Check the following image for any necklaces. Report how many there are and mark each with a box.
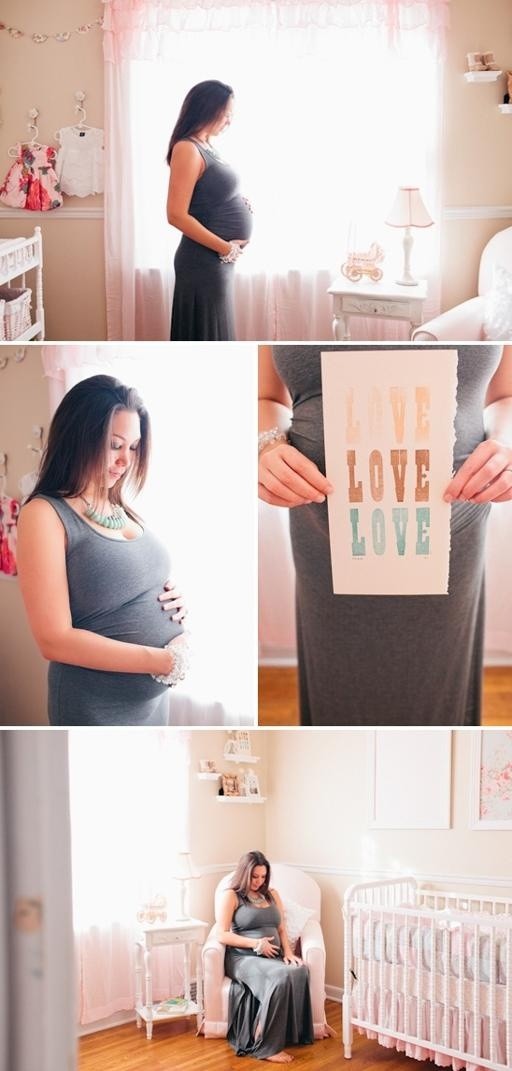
[246,892,267,905]
[77,487,129,530]
[194,134,224,161]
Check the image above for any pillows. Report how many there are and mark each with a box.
[280,892,315,953]
[483,261,512,340]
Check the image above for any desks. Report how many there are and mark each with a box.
[126,917,208,1040]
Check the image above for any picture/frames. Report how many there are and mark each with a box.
[367,731,454,828]
[469,730,512,832]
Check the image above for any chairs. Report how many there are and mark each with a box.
[411,219,512,340]
[201,863,329,1040]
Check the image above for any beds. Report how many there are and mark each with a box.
[339,875,512,1070]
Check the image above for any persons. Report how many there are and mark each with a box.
[257,345,512,726]
[14,372,190,726]
[164,76,254,341]
[215,850,311,1064]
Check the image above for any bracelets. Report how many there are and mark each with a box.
[256,423,283,449]
[149,642,186,686]
[252,939,265,955]
[217,242,244,267]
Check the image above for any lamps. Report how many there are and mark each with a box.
[385,183,437,287]
[170,852,200,920]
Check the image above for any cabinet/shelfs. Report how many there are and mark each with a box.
[463,69,512,115]
[0,225,44,342]
[325,281,430,340]
[197,753,268,804]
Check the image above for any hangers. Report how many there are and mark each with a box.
[53,106,91,141]
[0,472,19,515]
[17,446,43,491]
[6,125,56,159]
